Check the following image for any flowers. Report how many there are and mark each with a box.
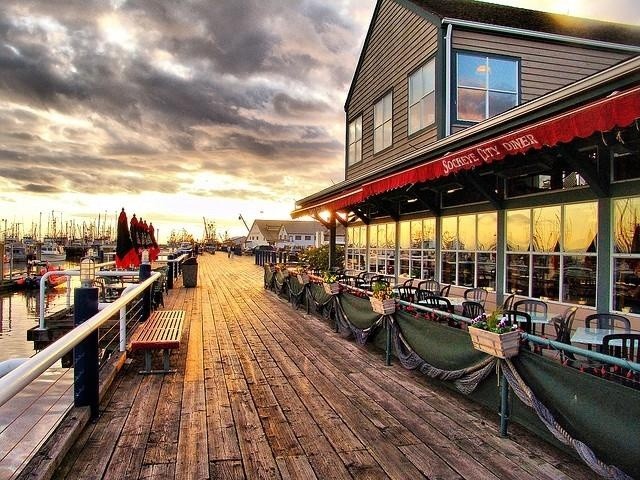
[298,268,304,274]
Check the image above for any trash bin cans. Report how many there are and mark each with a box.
[181,258,198,288]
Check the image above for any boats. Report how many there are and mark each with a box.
[0,210,117,295]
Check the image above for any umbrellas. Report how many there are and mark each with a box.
[116,207,160,287]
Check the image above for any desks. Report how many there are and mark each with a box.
[569,325,639,352]
[418,297,481,306]
[496,310,550,333]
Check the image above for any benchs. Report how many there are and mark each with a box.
[132,309,185,375]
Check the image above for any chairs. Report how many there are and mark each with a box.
[512,298,549,336]
[540,306,591,370]
[301,263,454,315]
[583,312,631,353]
[600,333,640,384]
[501,309,533,341]
[460,300,486,321]
[501,294,514,311]
[464,287,489,308]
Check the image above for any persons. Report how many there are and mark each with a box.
[27,249,36,276]
[271,243,276,251]
[227,244,236,259]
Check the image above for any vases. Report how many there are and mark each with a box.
[296,274,309,285]
